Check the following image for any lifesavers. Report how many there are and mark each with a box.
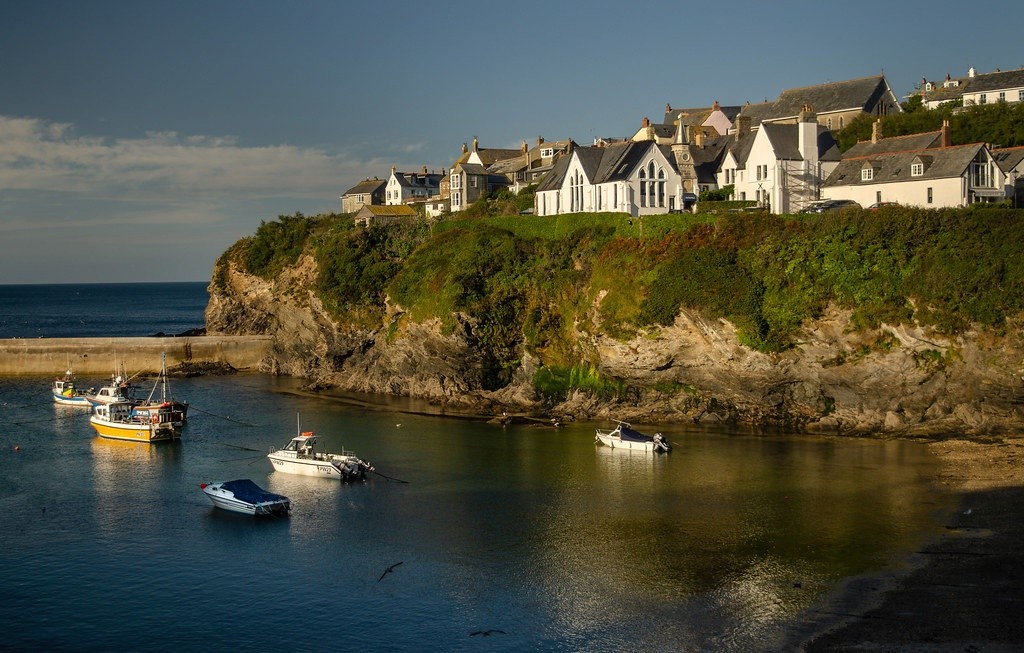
[301,431,314,436]
[151,413,158,424]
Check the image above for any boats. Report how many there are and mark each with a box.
[202,477,292,516]
[90,352,188,442]
[52,351,96,406]
[85,349,131,406]
[105,349,130,396]
[595,418,672,452]
[268,412,375,481]
